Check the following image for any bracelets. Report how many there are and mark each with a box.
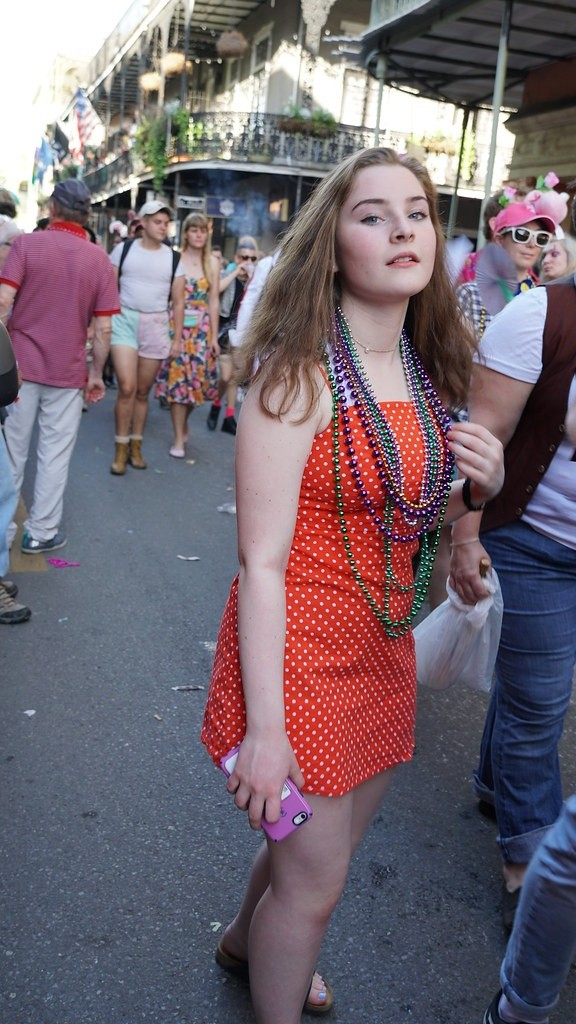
[462,478,482,511]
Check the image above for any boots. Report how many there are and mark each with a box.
[110,435,130,474]
[129,433,147,469]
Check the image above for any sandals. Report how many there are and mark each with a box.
[213,928,334,1013]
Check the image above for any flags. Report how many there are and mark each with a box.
[76,87,105,147]
[32,121,71,184]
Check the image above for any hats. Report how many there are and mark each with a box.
[492,185,556,245]
[53,180,90,211]
[139,201,176,221]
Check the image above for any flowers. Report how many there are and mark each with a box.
[497,172,558,213]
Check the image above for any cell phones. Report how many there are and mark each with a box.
[220,746,312,842]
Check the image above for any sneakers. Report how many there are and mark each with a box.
[21,531,67,553]
[0,578,32,624]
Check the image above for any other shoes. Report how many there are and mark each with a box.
[82,402,89,412]
[169,443,185,458]
[102,375,117,390]
[221,417,237,436]
[159,396,170,410]
[206,404,222,430]
[500,868,521,933]
[482,988,531,1024]
[476,797,498,824]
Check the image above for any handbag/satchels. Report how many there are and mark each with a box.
[411,564,504,693]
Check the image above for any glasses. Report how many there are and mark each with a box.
[497,225,552,249]
[237,254,257,262]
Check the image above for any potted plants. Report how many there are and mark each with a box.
[281,105,338,138]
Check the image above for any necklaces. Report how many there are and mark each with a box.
[314,308,459,639]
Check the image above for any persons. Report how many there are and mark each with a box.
[203,144,507,1017]
[453,177,576,959]
[0,179,121,552]
[0,189,279,475]
[1,316,32,626]
